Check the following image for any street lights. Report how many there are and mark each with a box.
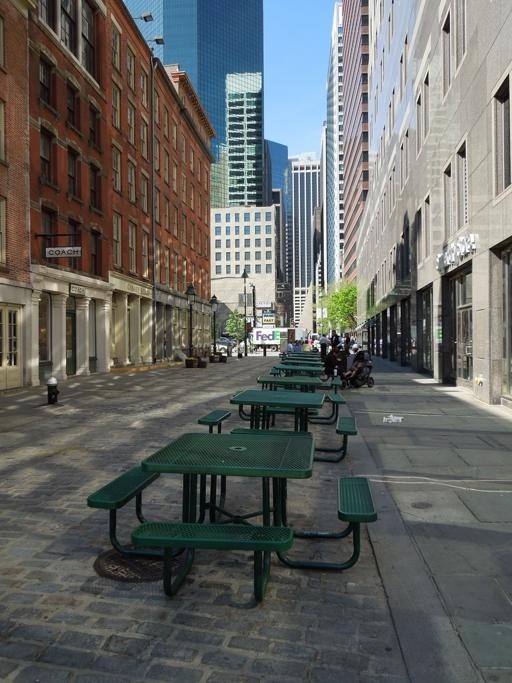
[240,268,251,356]
[184,282,197,358]
[209,294,219,356]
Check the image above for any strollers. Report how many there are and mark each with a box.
[340,350,376,389]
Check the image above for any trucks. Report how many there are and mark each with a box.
[253,328,318,351]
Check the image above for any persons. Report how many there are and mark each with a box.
[287,330,364,380]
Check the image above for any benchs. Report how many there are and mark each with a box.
[198,407,232,434]
[86,431,204,546]
[132,522,291,602]
[283,375,378,569]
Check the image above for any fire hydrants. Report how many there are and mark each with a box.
[47,377,60,405]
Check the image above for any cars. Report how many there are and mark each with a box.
[217,337,234,352]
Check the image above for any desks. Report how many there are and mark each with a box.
[231,388,325,436]
[258,376,323,392]
[274,352,326,372]
[144,432,316,525]
